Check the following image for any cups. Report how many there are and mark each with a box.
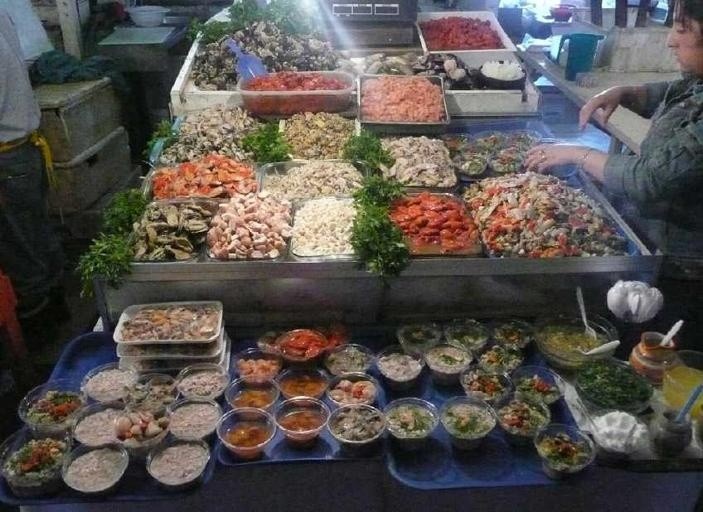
[661,351,703,416]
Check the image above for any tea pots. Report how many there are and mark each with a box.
[626,330,675,386]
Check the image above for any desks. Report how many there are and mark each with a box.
[515,39,682,157]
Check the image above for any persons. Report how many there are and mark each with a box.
[521,0,701,312]
[0,0,76,361]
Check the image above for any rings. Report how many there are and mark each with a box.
[537,150,545,154]
[538,155,550,162]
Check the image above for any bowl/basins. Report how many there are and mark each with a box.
[219,328,383,458]
[573,357,655,415]
[443,319,492,351]
[536,311,619,368]
[382,397,439,440]
[550,5,576,22]
[121,5,171,28]
[510,365,568,405]
[0,359,231,493]
[587,408,647,455]
[427,341,474,377]
[459,362,510,399]
[442,126,579,182]
[493,316,536,351]
[533,425,597,480]
[395,321,443,355]
[498,391,553,442]
[377,345,426,381]
[439,395,498,441]
[474,347,526,374]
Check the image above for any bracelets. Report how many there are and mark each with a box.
[577,144,592,170]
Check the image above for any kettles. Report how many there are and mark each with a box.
[554,34,606,83]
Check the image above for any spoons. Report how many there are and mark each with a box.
[574,286,597,342]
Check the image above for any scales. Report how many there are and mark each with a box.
[327,1,416,48]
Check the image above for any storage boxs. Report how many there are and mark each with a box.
[97,26,176,72]
[32,77,143,240]
